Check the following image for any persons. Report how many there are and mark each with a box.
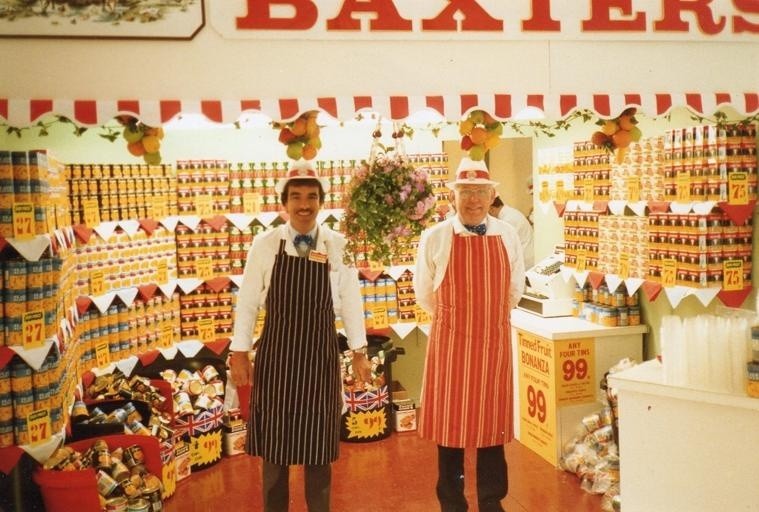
[412,159,526,512]
[487,192,535,273]
[231,162,372,511]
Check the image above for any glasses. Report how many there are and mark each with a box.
[459,188,491,198]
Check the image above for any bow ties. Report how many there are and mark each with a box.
[464,223,486,236]
[294,234,314,248]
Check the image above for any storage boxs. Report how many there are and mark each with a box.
[393,399,416,432]
[392,380,407,399]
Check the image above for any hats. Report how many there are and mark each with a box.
[445,157,500,188]
[275,164,330,195]
[490,192,499,206]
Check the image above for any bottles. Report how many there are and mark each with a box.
[48,157,65,193]
[5,262,27,347]
[15,194,31,203]
[46,341,84,434]
[43,196,71,233]
[681,319,708,388]
[180,160,228,211]
[64,164,178,225]
[727,319,747,392]
[11,363,34,445]
[231,162,368,209]
[564,122,759,302]
[157,365,225,418]
[660,316,683,378]
[175,222,398,340]
[56,230,179,378]
[398,157,454,319]
[557,357,636,494]
[31,193,48,234]
[0,370,14,447]
[572,270,641,327]
[0,193,15,238]
[11,151,31,193]
[43,439,163,512]
[0,150,14,192]
[710,318,727,391]
[72,372,176,441]
[30,152,49,192]
[39,259,55,337]
[340,349,385,392]
[24,262,43,310]
[26,364,51,409]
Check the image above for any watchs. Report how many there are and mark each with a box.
[353,346,369,355]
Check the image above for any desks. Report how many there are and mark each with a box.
[509,308,650,468]
[607,356,759,512]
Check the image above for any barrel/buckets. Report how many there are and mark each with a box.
[72,401,151,434]
[340,335,406,443]
[81,380,175,500]
[33,435,162,512]
[121,358,226,472]
